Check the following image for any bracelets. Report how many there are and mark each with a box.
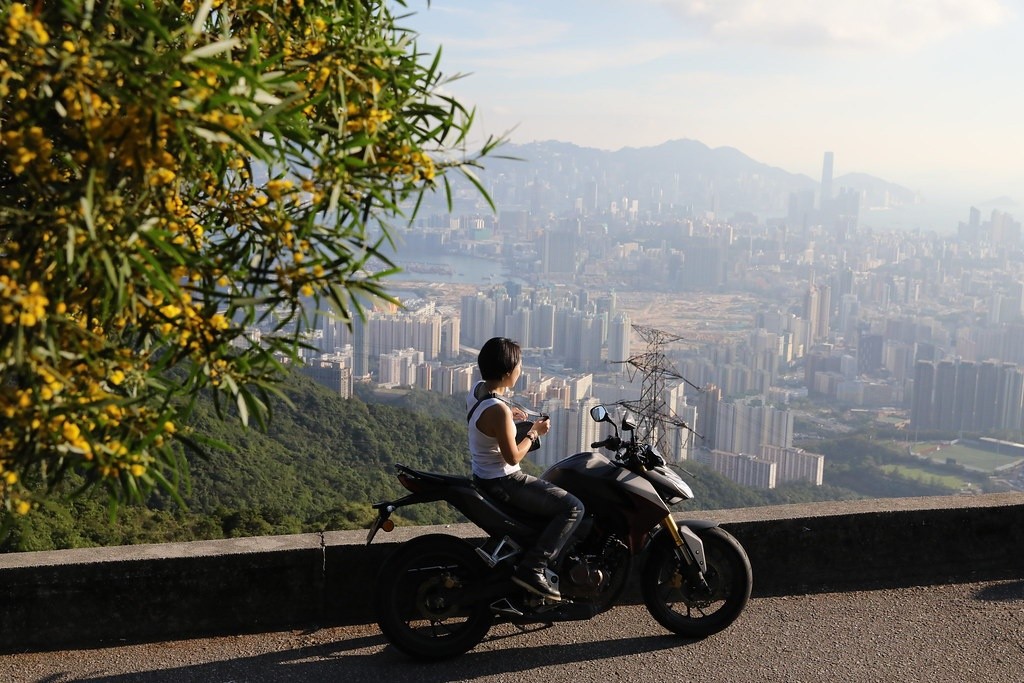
[527,430,538,440]
[526,434,533,440]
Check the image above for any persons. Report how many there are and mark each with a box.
[466,337,585,600]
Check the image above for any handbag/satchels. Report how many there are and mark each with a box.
[515,420,540,453]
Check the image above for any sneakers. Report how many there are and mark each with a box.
[511,564,562,600]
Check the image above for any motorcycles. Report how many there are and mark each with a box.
[366,405,753,663]
